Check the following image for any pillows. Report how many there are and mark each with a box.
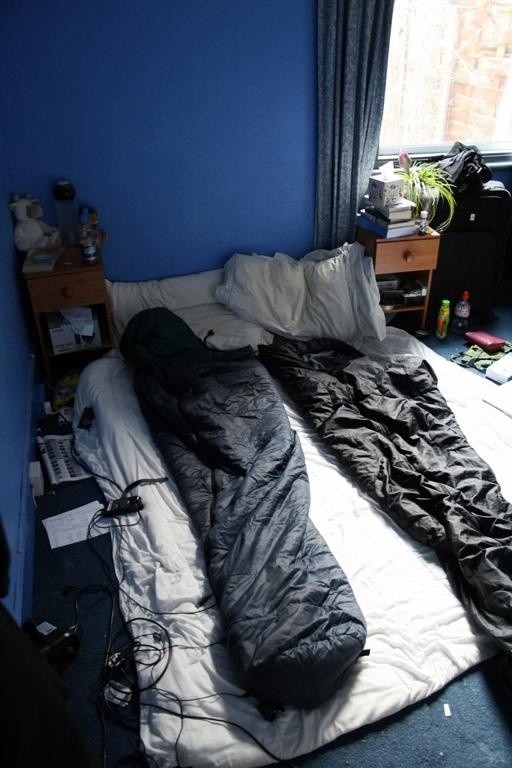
[104,268,274,360]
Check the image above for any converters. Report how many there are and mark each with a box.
[104,496,141,518]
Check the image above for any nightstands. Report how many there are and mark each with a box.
[357,226,440,330]
[23,244,115,386]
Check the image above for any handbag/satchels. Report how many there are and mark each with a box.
[461,331,512,366]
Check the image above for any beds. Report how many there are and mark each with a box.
[73,243,512,768]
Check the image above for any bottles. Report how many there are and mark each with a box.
[53,179,100,264]
[436,289,472,339]
[104,650,140,708]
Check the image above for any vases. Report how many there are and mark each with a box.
[404,188,439,227]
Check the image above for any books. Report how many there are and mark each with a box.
[33,431,93,485]
[354,192,418,241]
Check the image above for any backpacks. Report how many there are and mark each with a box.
[433,141,492,211]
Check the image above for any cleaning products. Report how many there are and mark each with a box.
[452,291,470,335]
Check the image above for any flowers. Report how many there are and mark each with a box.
[393,153,457,232]
